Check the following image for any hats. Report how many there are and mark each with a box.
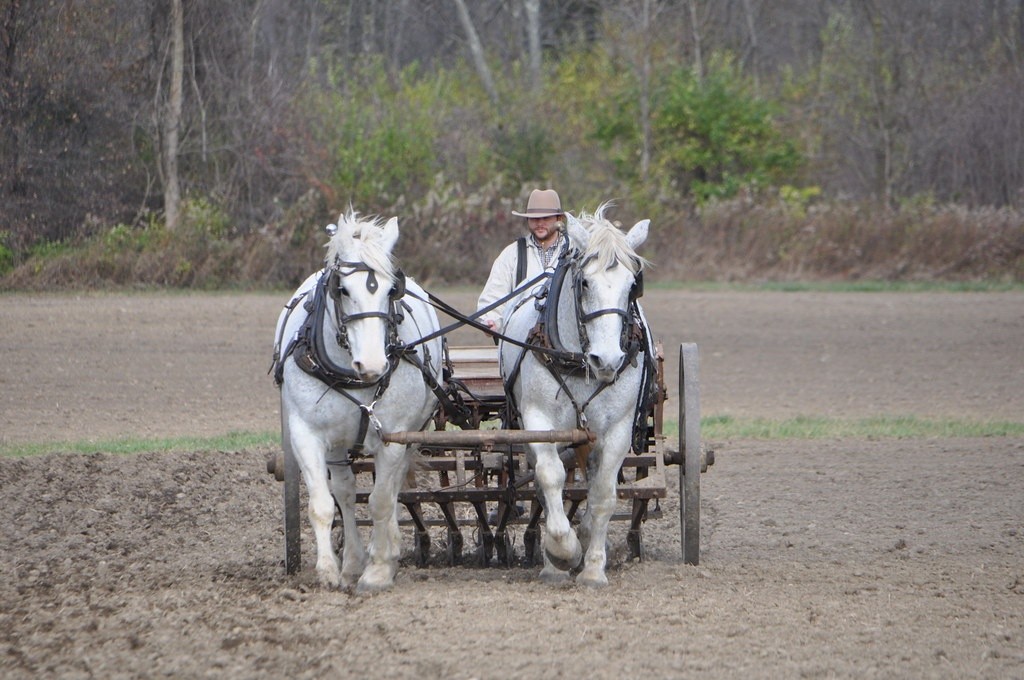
[512,189,575,219]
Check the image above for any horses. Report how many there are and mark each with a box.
[273,202,443,593]
[501,198,658,588]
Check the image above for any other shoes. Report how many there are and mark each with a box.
[489,504,524,521]
[564,500,584,521]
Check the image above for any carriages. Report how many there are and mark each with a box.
[264,208,717,594]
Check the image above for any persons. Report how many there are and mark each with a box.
[476,189,584,526]
